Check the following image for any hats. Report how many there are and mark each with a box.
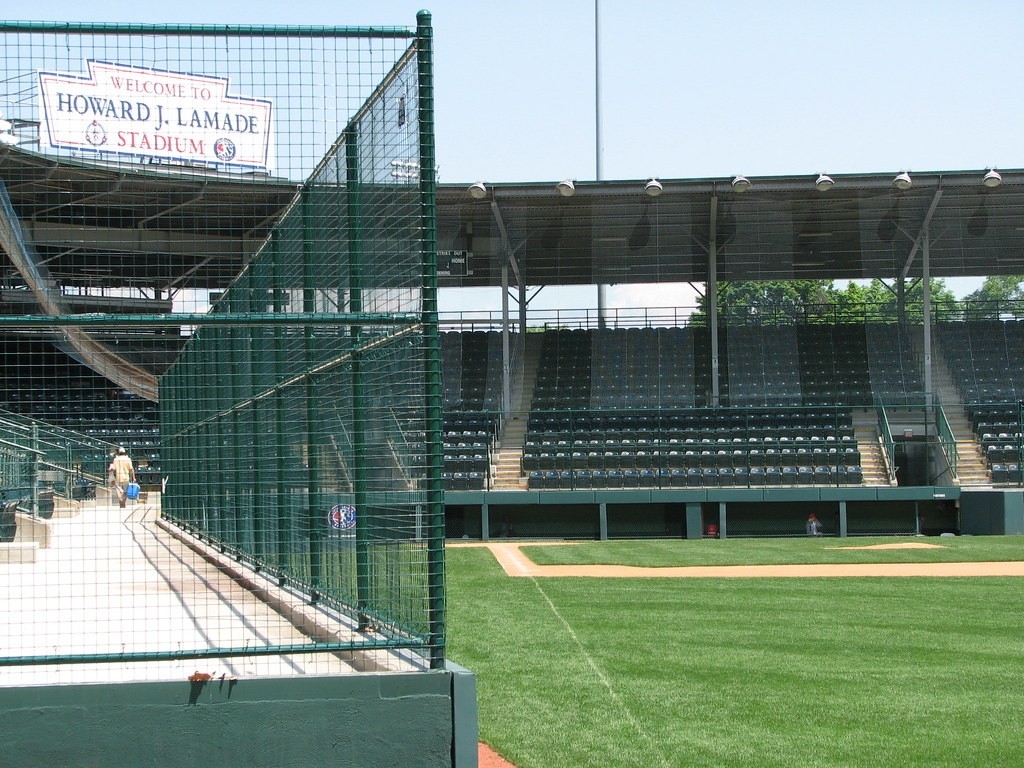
[119,447,125,452]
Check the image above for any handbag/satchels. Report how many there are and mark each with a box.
[109,472,116,487]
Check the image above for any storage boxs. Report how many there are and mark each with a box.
[126,482,141,499]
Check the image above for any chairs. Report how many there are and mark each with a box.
[0,316,1024,493]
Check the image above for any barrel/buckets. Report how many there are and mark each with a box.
[126,484,140,498]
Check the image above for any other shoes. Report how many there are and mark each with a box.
[119,501,125,508]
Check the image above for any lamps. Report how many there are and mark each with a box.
[467,180,487,198]
[644,177,663,196]
[815,173,833,192]
[982,166,1001,188]
[731,175,750,193]
[893,169,911,190]
[554,180,577,198]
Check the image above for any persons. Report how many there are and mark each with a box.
[108,447,136,508]
